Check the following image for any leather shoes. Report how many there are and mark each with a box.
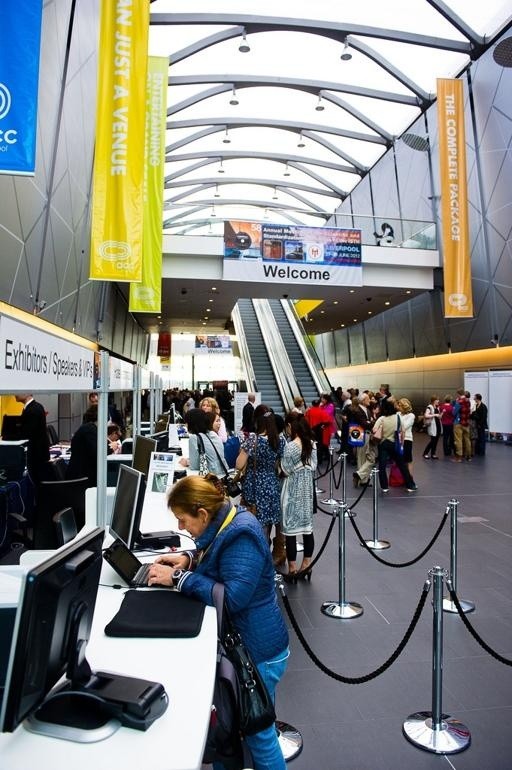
[407,489,416,493]
[353,473,360,488]
[361,483,372,488]
[382,489,390,493]
[423,454,472,462]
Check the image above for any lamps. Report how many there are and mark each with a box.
[215,28,352,180]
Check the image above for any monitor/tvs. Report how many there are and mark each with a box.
[133,414,170,477]
[0,527,170,744]
[109,463,180,552]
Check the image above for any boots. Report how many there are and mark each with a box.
[271,535,286,567]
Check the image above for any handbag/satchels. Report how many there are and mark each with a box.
[239,499,256,515]
[394,415,404,456]
[423,407,431,428]
[344,421,366,447]
[212,583,276,736]
[222,476,243,498]
[389,463,406,487]
[197,433,209,478]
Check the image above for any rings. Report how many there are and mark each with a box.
[153,578,159,583]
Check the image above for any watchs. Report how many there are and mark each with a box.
[172,569,185,586]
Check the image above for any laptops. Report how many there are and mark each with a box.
[103,538,181,592]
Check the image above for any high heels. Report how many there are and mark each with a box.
[297,568,312,582]
[285,569,298,585]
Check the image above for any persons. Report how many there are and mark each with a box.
[293,384,419,494]
[235,394,318,582]
[147,474,291,770]
[74,388,241,482]
[421,388,487,463]
[1,394,51,466]
[373,223,394,246]
